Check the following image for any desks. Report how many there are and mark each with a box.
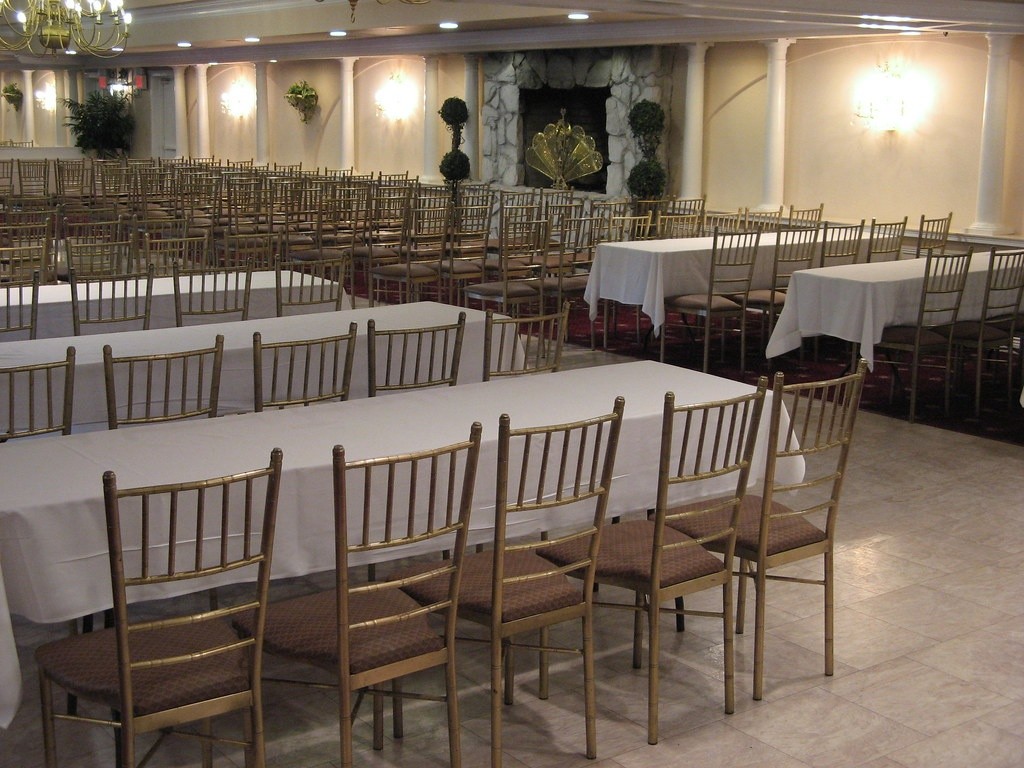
[584,225,906,368]
[0,296,529,444]
[0,265,336,344]
[0,356,808,730]
[765,244,1022,413]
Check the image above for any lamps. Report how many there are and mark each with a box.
[0,0,132,57]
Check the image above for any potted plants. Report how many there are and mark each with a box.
[285,80,319,123]
[3,83,23,111]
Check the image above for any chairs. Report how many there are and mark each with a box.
[0,139,1023,767]
